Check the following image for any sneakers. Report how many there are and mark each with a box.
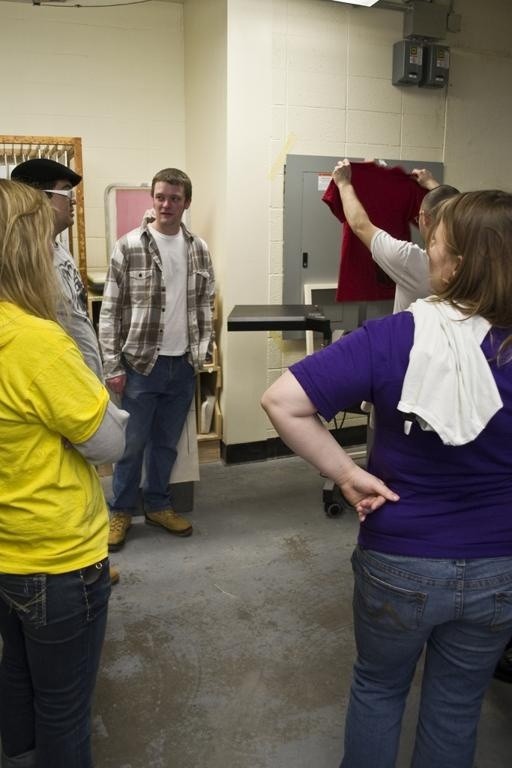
[108,511,132,550]
[145,510,193,537]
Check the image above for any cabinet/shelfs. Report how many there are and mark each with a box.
[86,287,222,468]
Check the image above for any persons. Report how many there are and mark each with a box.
[257,185,512,767]
[9,157,122,588]
[97,166,217,554]
[330,157,463,316]
[0,177,133,768]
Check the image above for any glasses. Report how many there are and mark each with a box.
[41,190,73,199]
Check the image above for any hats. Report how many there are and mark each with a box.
[11,158,82,187]
